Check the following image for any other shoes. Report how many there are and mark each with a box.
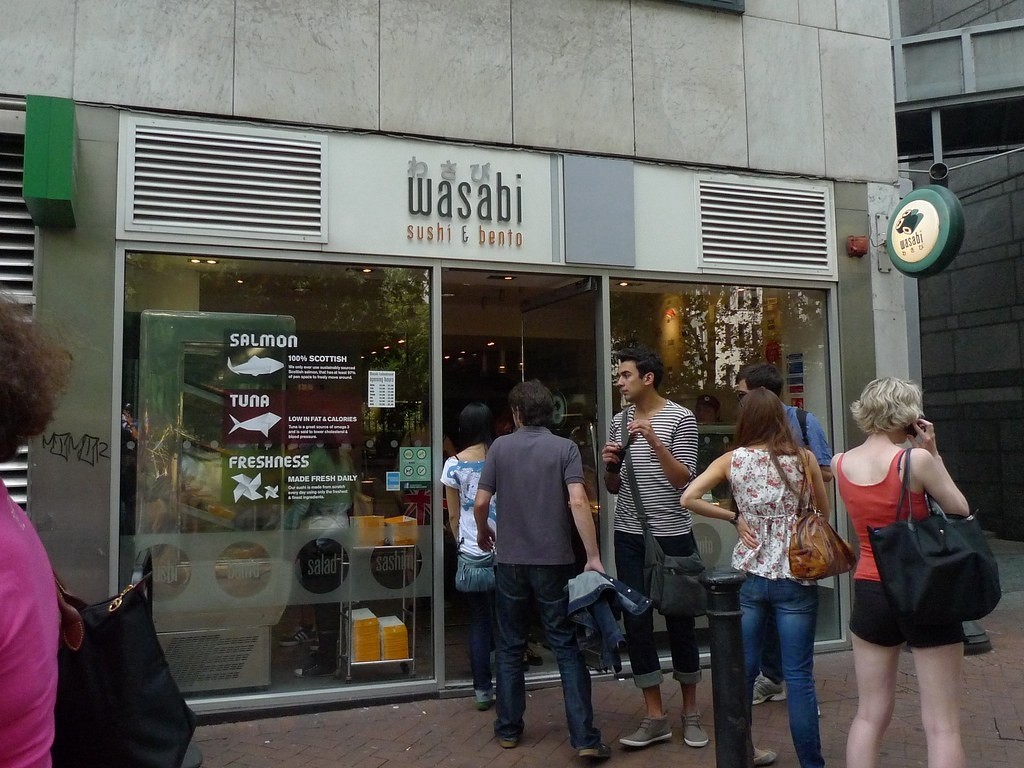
[753,744,777,765]
[476,694,496,711]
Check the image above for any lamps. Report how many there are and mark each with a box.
[518,350,526,372]
[498,349,508,373]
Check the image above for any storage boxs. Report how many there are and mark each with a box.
[349,514,386,547]
[384,515,420,546]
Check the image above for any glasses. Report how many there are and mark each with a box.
[613,416,639,454]
[736,393,746,402]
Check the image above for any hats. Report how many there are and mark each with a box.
[696,394,721,410]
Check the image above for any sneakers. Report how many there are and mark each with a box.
[619,711,672,747]
[680,708,709,747]
[526,646,544,666]
[752,670,787,705]
[520,652,530,671]
[578,743,612,759]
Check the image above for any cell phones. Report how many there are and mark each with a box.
[905,422,921,439]
[606,448,628,474]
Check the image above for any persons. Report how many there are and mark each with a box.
[829,376,970,768]
[264,398,545,712]
[0,288,75,767]
[476,381,607,758]
[599,347,711,747]
[679,362,830,768]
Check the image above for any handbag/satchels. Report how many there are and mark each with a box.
[788,447,858,580]
[643,525,709,618]
[455,551,497,592]
[866,448,1003,639]
[51,563,200,768]
[349,491,374,517]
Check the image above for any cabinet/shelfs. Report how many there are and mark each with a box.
[333,543,419,686]
